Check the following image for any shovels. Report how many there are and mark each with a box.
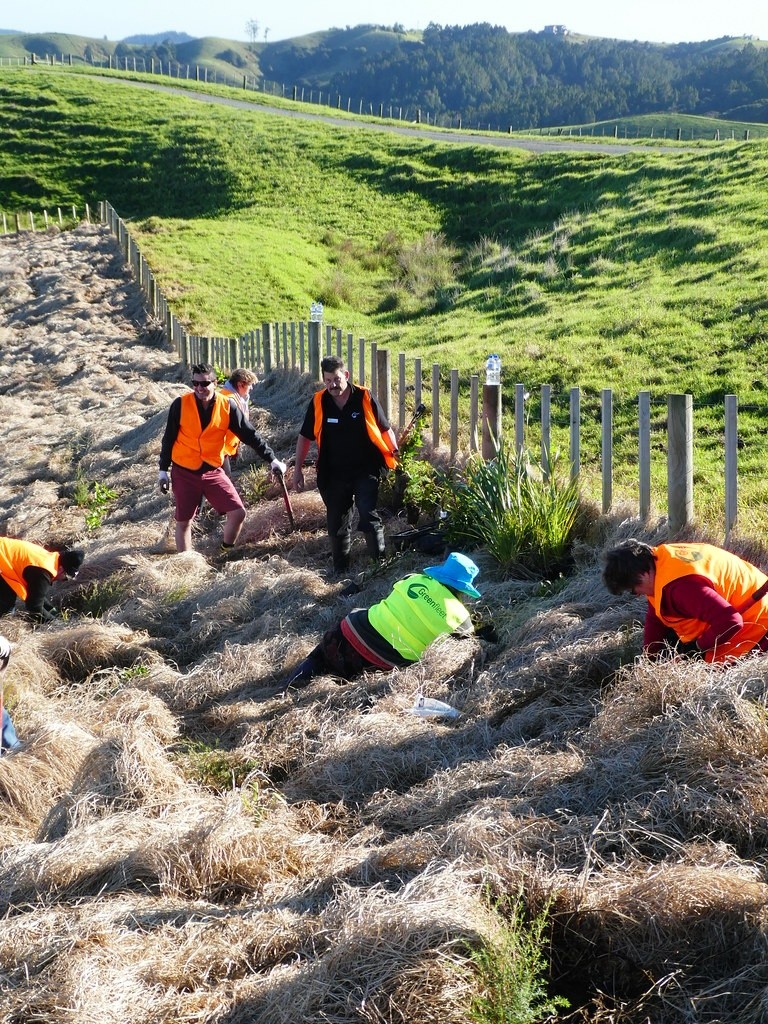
[340,540,419,597]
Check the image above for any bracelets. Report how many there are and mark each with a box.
[391,449,398,456]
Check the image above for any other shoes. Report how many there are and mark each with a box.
[220,544,234,554]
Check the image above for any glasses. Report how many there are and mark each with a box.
[191,378,215,387]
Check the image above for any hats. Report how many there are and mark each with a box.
[59,551,85,580]
[422,552,482,600]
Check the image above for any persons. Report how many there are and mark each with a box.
[292,355,401,580]
[602,538,768,673]
[0,537,84,626]
[278,552,483,690]
[0,636,22,757]
[158,363,287,555]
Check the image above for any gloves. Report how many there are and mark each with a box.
[270,459,286,483]
[159,471,170,494]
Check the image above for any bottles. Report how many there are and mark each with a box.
[316,302,323,322]
[310,302,316,322]
[486,354,501,385]
